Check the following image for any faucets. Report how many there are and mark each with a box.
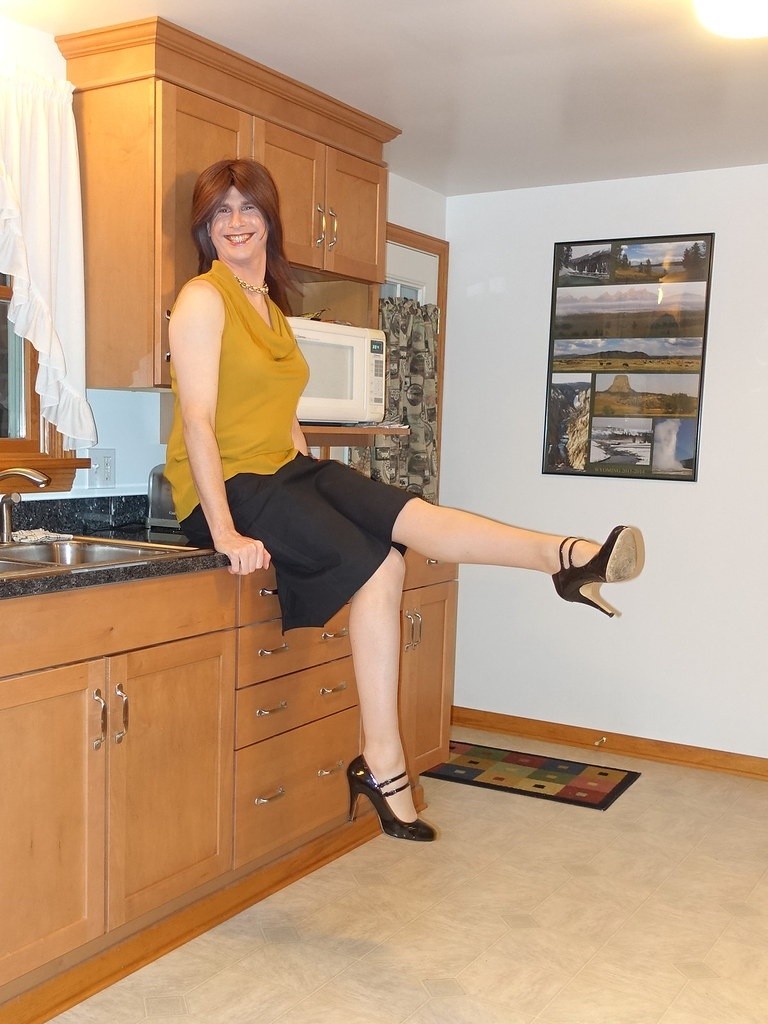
[0,467,53,489]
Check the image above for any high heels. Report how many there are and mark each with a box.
[346,752,437,842]
[552,525,637,619]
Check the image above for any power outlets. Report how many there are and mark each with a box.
[87,449,116,489]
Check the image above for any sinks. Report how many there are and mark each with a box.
[0,540,168,566]
[0,560,53,574]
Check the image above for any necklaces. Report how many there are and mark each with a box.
[233,273,268,295]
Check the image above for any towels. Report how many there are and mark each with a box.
[11,527,75,545]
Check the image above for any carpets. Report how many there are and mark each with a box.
[420,739,641,813]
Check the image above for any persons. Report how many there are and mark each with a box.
[165,159,644,842]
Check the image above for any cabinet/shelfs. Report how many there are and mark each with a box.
[0,541,456,984]
[73,73,388,393]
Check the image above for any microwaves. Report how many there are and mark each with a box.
[284,316,386,424]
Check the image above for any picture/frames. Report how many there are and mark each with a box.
[539,232,716,485]
